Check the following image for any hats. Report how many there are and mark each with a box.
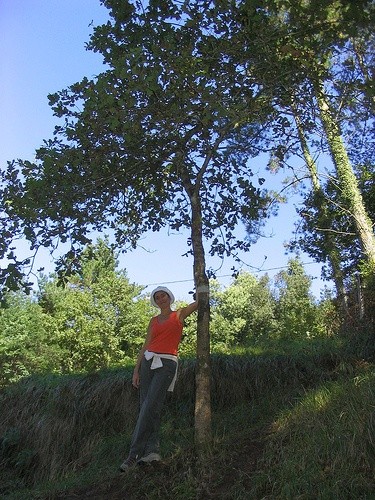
[150,286,174,307]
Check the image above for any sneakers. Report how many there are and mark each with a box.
[137,453,160,466]
[120,456,137,473]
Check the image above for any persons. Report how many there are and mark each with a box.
[120,285,198,473]
